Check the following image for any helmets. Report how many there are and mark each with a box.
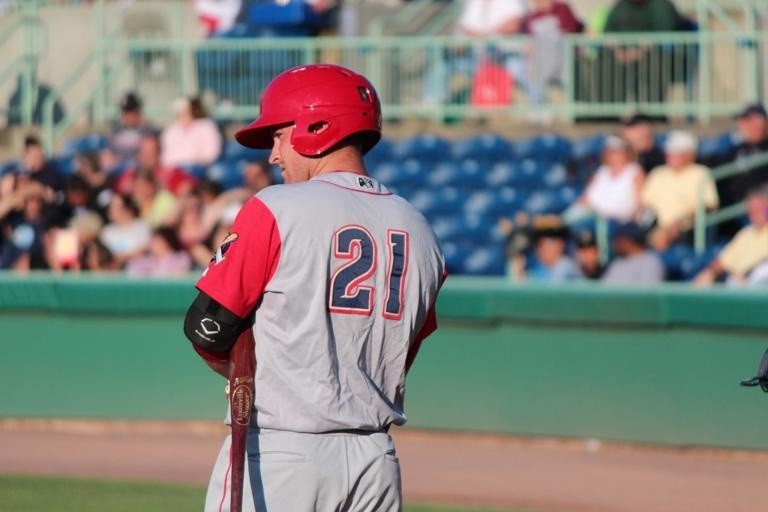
[235,63,382,157]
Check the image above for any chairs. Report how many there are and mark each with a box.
[367,130,733,273]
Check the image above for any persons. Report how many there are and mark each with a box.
[195,0,697,106]
[1,71,271,273]
[497,103,768,290]
[184,66,447,512]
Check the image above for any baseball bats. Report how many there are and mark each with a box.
[228,330,256,511]
